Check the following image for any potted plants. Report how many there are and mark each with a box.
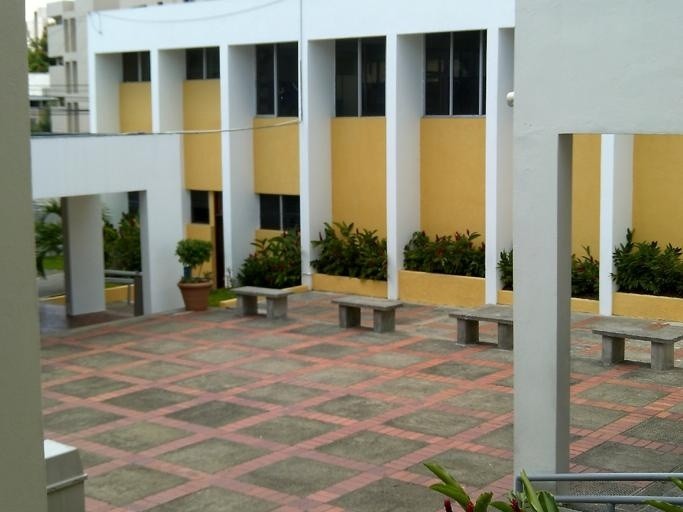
[175,236,214,311]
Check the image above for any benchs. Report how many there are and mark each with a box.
[447,304,514,350]
[229,285,295,319]
[330,295,404,334]
[588,318,683,373]
